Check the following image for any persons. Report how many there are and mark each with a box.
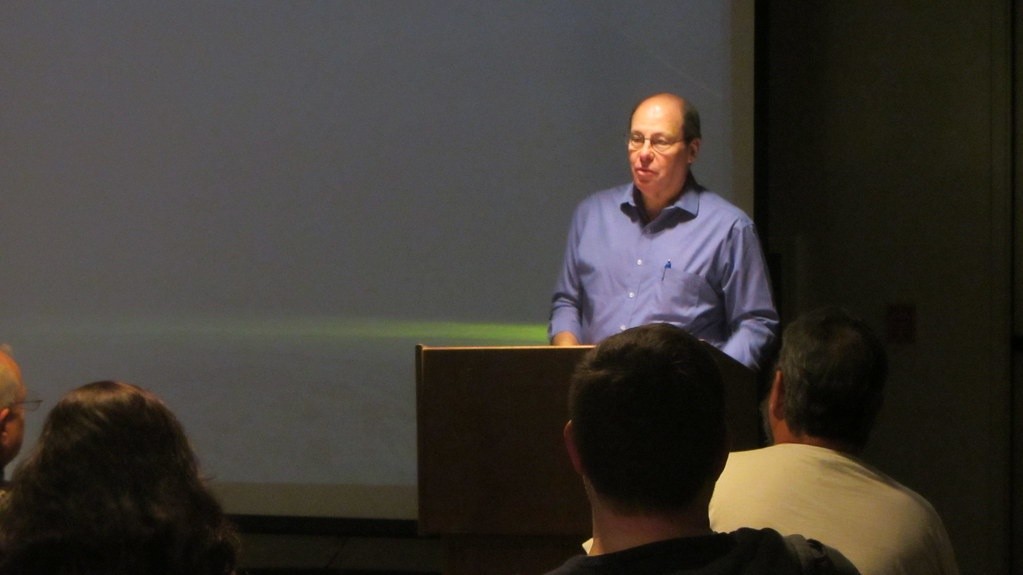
[548,92,780,371]
[0,380,242,575]
[545,323,859,575]
[0,344,44,510]
[708,309,960,575]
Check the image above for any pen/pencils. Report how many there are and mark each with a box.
[661,260,671,279]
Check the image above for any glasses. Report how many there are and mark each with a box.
[622,133,685,153]
[8,390,43,411]
